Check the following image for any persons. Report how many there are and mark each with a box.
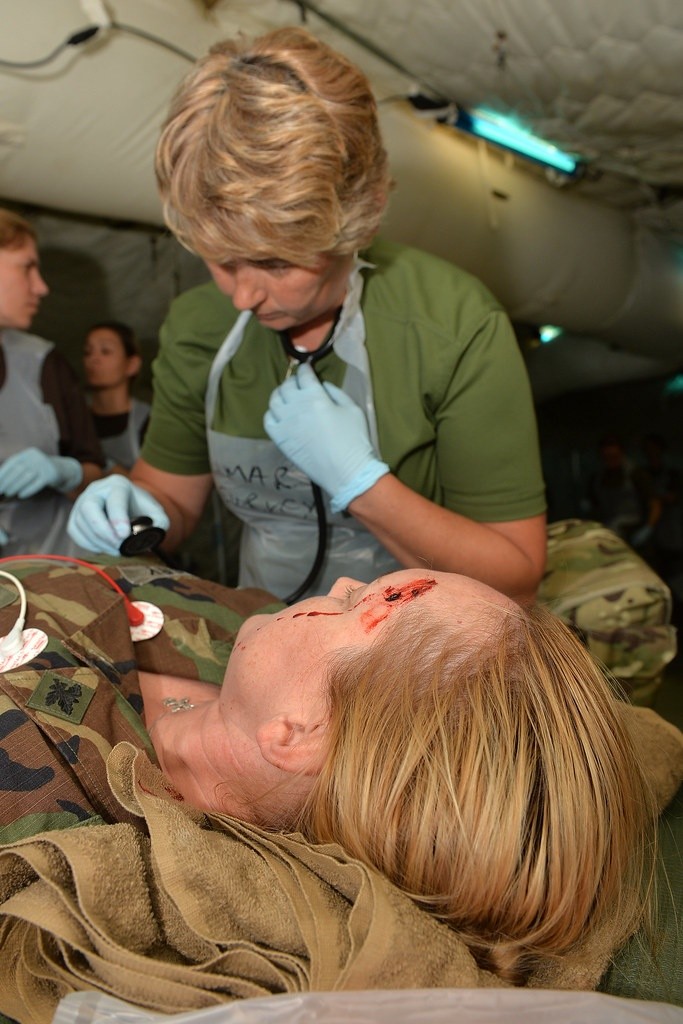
[84,322,151,474]
[589,441,663,568]
[68,29,545,608]
[0,209,106,557]
[642,435,683,577]
[0,553,662,949]
